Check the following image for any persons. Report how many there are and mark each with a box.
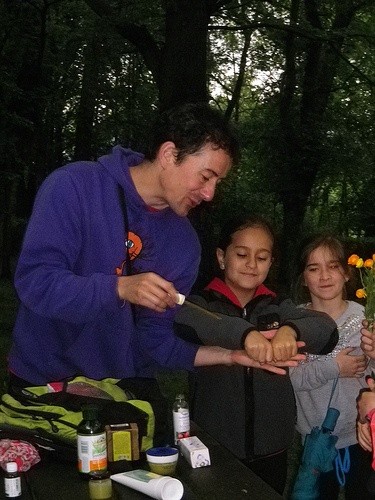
[7,102,307,397]
[289,235,375,500]
[173,212,339,497]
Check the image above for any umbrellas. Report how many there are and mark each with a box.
[291,408,340,500]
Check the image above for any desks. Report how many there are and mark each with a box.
[0,413,283,500]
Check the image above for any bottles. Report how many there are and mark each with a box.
[172,393,190,447]
[146,445,179,476]
[4,461,21,499]
[88,470,113,499]
[77,409,108,478]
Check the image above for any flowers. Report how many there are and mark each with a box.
[348,254,375,332]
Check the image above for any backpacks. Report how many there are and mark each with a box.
[0,376,155,468]
